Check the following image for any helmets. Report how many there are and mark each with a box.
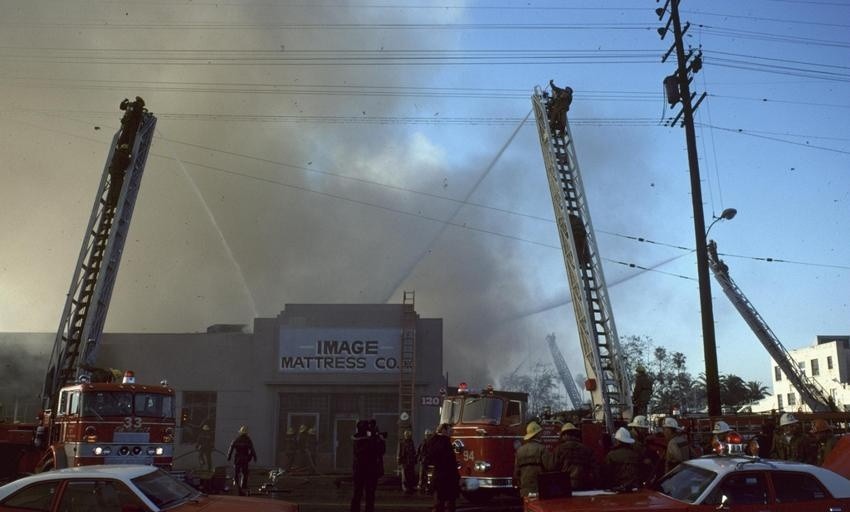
[561,422,581,434]
[238,424,248,434]
[401,412,409,421]
[404,431,413,439]
[712,421,734,434]
[809,419,830,433]
[203,425,210,431]
[627,415,650,429]
[523,421,543,441]
[780,413,799,426]
[636,366,645,373]
[110,368,123,381]
[286,424,317,436]
[614,427,635,444]
[662,417,682,431]
[425,430,433,435]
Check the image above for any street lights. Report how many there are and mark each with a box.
[705,208,737,239]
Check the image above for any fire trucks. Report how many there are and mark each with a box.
[535,334,594,426]
[437,89,769,505]
[0,96,233,494]
[675,243,850,463]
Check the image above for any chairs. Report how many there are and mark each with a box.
[45,488,124,512]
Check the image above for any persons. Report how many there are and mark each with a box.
[228,427,259,495]
[283,424,319,475]
[78,361,122,383]
[348,417,462,511]
[514,413,850,500]
[195,424,215,471]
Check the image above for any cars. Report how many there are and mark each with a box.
[0,464,301,512]
[524,433,850,511]
